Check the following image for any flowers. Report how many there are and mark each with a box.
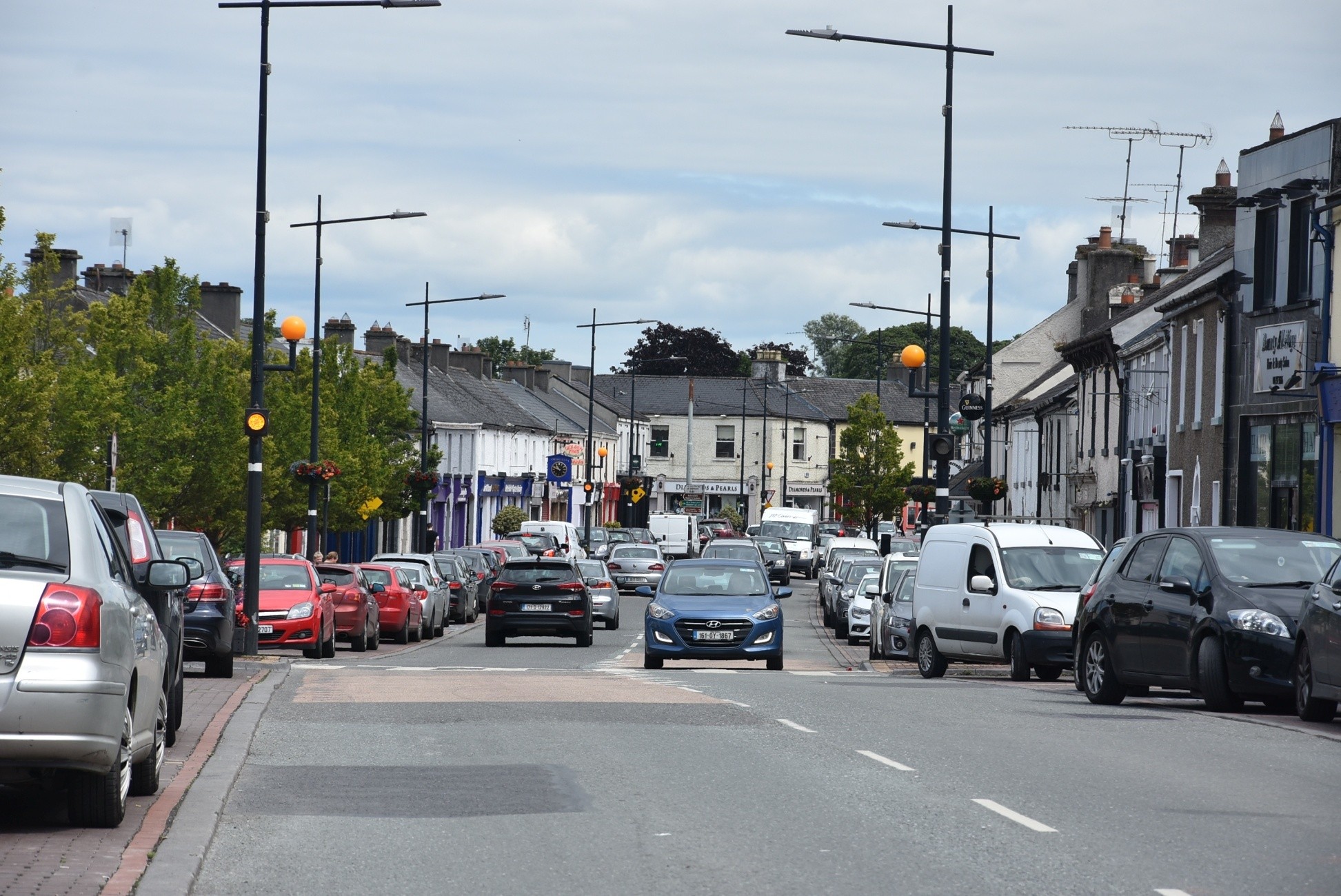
[907,485,930,496]
[620,477,644,485]
[964,477,1011,496]
[291,460,341,480]
[404,471,440,485]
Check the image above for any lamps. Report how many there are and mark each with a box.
[1285,365,1341,390]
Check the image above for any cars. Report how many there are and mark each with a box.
[150,529,243,681]
[1069,525,1339,724]
[87,486,206,752]
[635,558,795,671]
[0,472,194,827]
[221,504,945,680]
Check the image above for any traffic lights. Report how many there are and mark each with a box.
[930,433,956,459]
[584,481,594,494]
[630,454,641,467]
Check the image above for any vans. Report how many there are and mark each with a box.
[907,521,1114,683]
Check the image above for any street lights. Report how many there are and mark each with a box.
[403,281,507,554]
[617,354,690,476]
[738,376,775,525]
[287,195,427,561]
[781,5,997,537]
[782,386,814,508]
[577,307,660,556]
[882,206,1020,519]
[823,327,898,544]
[759,370,805,518]
[848,293,942,544]
[216,0,442,660]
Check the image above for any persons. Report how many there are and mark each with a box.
[795,502,799,508]
[426,522,440,554]
[313,551,323,563]
[726,575,753,592]
[895,509,905,536]
[804,503,811,509]
[941,515,949,524]
[323,552,339,563]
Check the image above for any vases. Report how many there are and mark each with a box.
[624,485,639,489]
[972,490,1004,501]
[912,495,922,502]
[297,476,331,485]
[410,481,436,489]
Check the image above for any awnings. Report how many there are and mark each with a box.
[948,459,985,500]
